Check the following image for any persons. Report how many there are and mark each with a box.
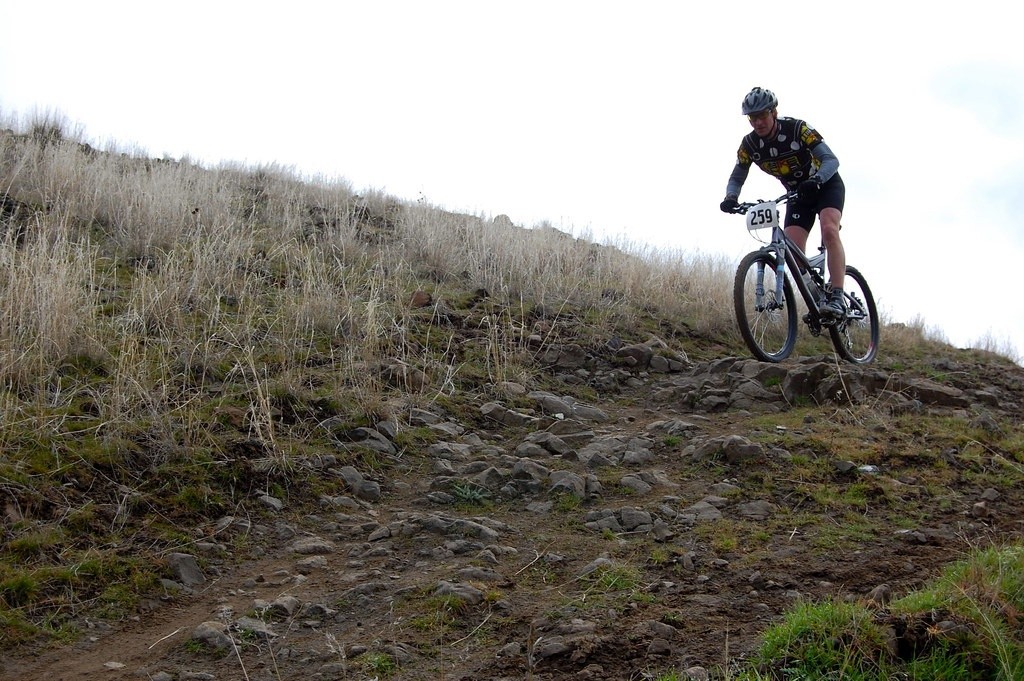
[723,87,852,318]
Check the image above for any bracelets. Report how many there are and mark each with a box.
[808,176,823,185]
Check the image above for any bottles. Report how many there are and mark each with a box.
[799,266,822,303]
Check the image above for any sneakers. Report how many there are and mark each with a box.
[821,303,847,315]
[802,314,808,323]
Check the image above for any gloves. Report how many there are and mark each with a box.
[721,199,738,214]
[798,180,821,201]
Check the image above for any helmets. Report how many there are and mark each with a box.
[742,89,778,115]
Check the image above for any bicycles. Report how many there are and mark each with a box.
[724,183,880,368]
